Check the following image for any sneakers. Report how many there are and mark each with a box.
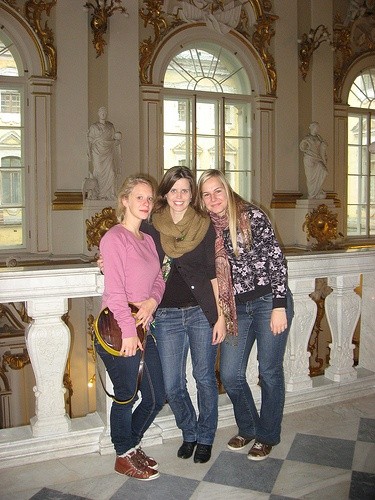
[177,440,197,460]
[134,445,160,471]
[226,433,256,450]
[194,443,212,463]
[114,448,160,482]
[247,441,272,461]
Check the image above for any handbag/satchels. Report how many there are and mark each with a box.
[94,302,148,357]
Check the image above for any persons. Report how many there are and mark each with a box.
[98,175,172,482]
[198,167,293,461]
[298,121,330,200]
[97,164,229,462]
